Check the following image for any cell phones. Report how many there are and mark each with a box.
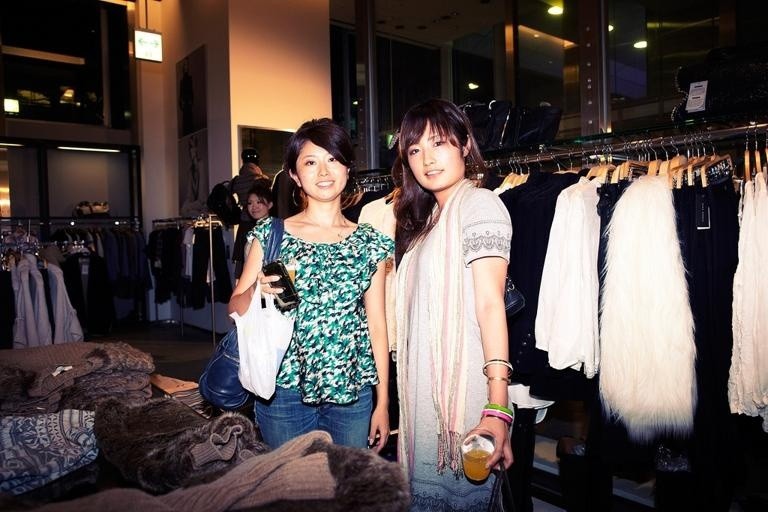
[262,260,299,305]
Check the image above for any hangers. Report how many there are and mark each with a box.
[0,217,139,272]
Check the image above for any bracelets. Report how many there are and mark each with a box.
[486,376,511,385]
[482,358,514,376]
[481,403,515,425]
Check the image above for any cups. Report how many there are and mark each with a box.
[458,428,497,483]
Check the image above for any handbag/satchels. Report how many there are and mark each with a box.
[505,276,526,320]
[72,200,114,220]
[198,324,249,411]
[205,181,242,227]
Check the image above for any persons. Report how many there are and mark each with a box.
[224,117,397,455]
[225,146,270,279]
[246,183,275,222]
[391,97,515,512]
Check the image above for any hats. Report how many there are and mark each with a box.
[241,148,260,163]
